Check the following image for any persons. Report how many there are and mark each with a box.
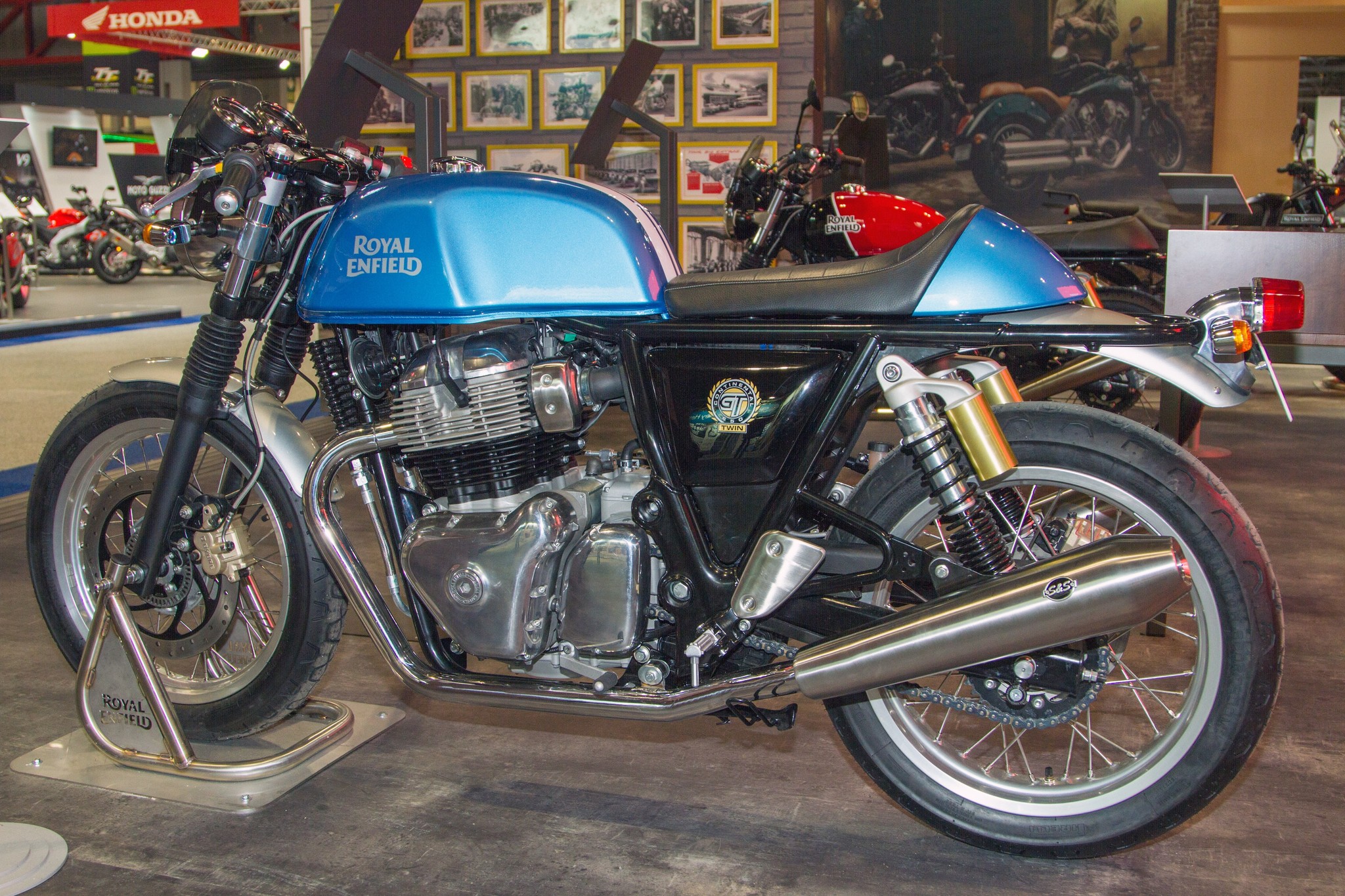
[557,79,567,120]
[484,12,509,29]
[657,1,673,40]
[1050,0,1119,64]
[842,0,891,192]
[648,75,664,92]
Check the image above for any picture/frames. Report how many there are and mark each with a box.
[360,0,780,275]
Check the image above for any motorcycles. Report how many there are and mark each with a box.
[1042,110,1344,300]
[0,192,37,311]
[950,10,1194,205]
[25,191,272,286]
[819,33,979,184]
[684,74,1205,451]
[19,77,1308,863]
